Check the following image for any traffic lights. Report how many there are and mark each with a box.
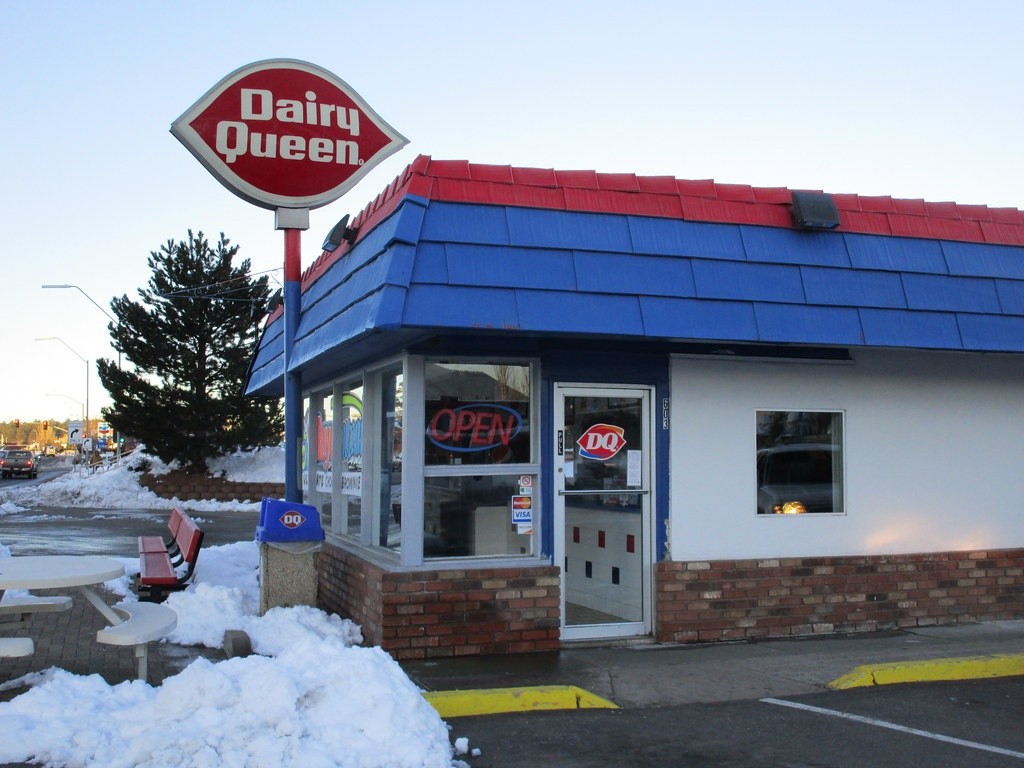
[15,419,19,427]
[43,421,47,430]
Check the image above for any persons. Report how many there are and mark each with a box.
[90,451,102,473]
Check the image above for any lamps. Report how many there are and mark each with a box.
[321,213,360,253]
[790,190,841,231]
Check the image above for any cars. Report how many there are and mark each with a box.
[0,446,55,479]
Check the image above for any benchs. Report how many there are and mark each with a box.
[138,506,205,598]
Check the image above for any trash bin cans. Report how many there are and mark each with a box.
[255,497,327,617]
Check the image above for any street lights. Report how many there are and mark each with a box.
[43,284,122,465]
[35,336,88,469]
